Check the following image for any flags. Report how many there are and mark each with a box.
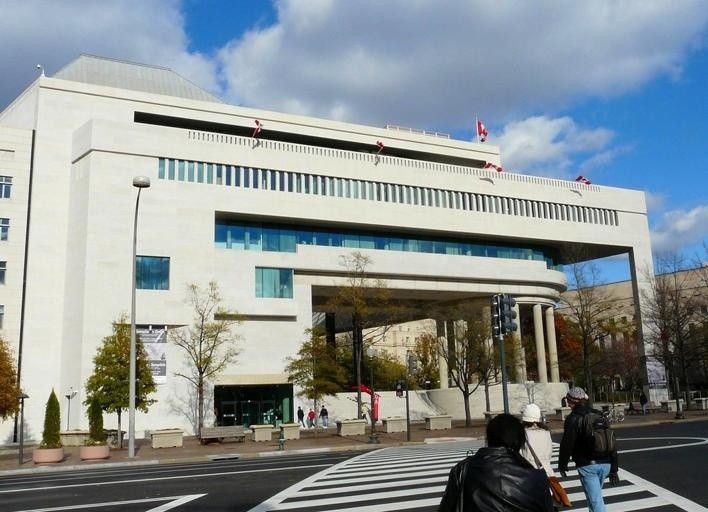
[476,118,488,142]
[576,175,591,185]
[485,162,502,173]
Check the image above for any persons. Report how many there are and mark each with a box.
[308,409,316,427]
[640,392,647,415]
[437,413,554,512]
[297,406,305,427]
[361,403,368,423]
[558,386,620,512]
[519,403,555,497]
[319,405,328,427]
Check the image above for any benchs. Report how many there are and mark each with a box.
[615,400,661,415]
[199,424,249,447]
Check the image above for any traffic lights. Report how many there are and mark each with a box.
[499,297,516,333]
[396,382,404,396]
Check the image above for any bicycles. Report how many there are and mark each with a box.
[603,407,624,423]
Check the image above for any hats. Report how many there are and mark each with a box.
[567,387,586,403]
[522,404,541,423]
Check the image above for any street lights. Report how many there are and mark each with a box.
[366,345,378,445]
[125,176,152,459]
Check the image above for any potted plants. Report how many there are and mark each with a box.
[76,397,113,460]
[32,386,67,465]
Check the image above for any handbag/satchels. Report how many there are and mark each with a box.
[548,476,571,507]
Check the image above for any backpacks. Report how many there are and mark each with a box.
[570,410,616,452]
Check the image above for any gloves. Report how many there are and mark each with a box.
[605,472,619,486]
[558,465,569,478]
